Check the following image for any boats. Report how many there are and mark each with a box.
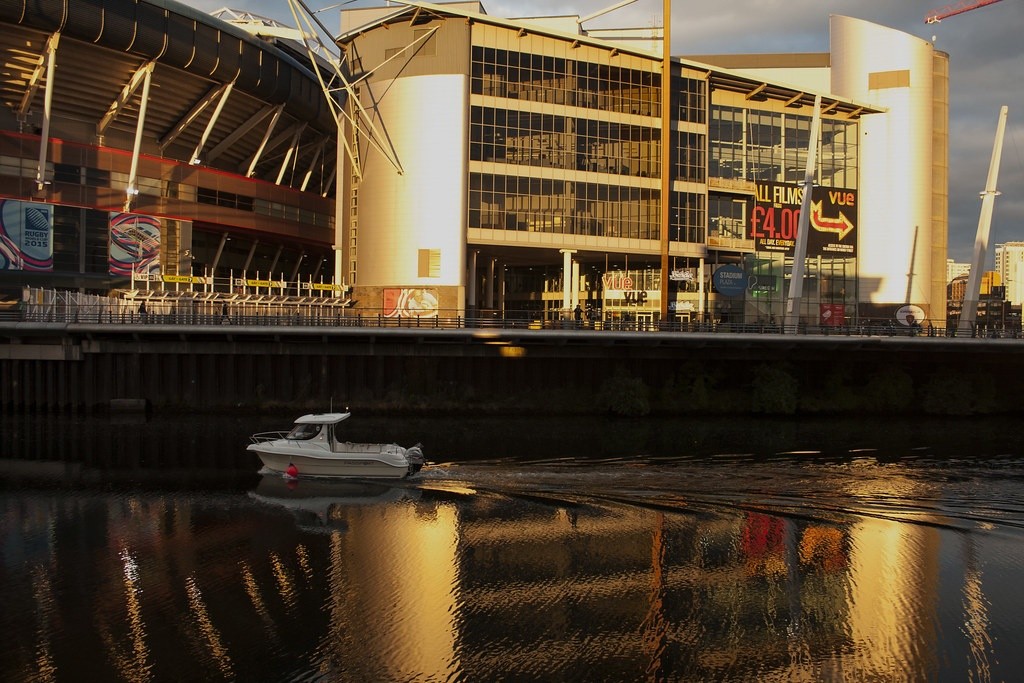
[246,398,427,481]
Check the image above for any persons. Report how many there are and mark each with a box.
[574,304,597,330]
[991,321,1001,339]
[220,301,233,325]
[136,300,149,324]
